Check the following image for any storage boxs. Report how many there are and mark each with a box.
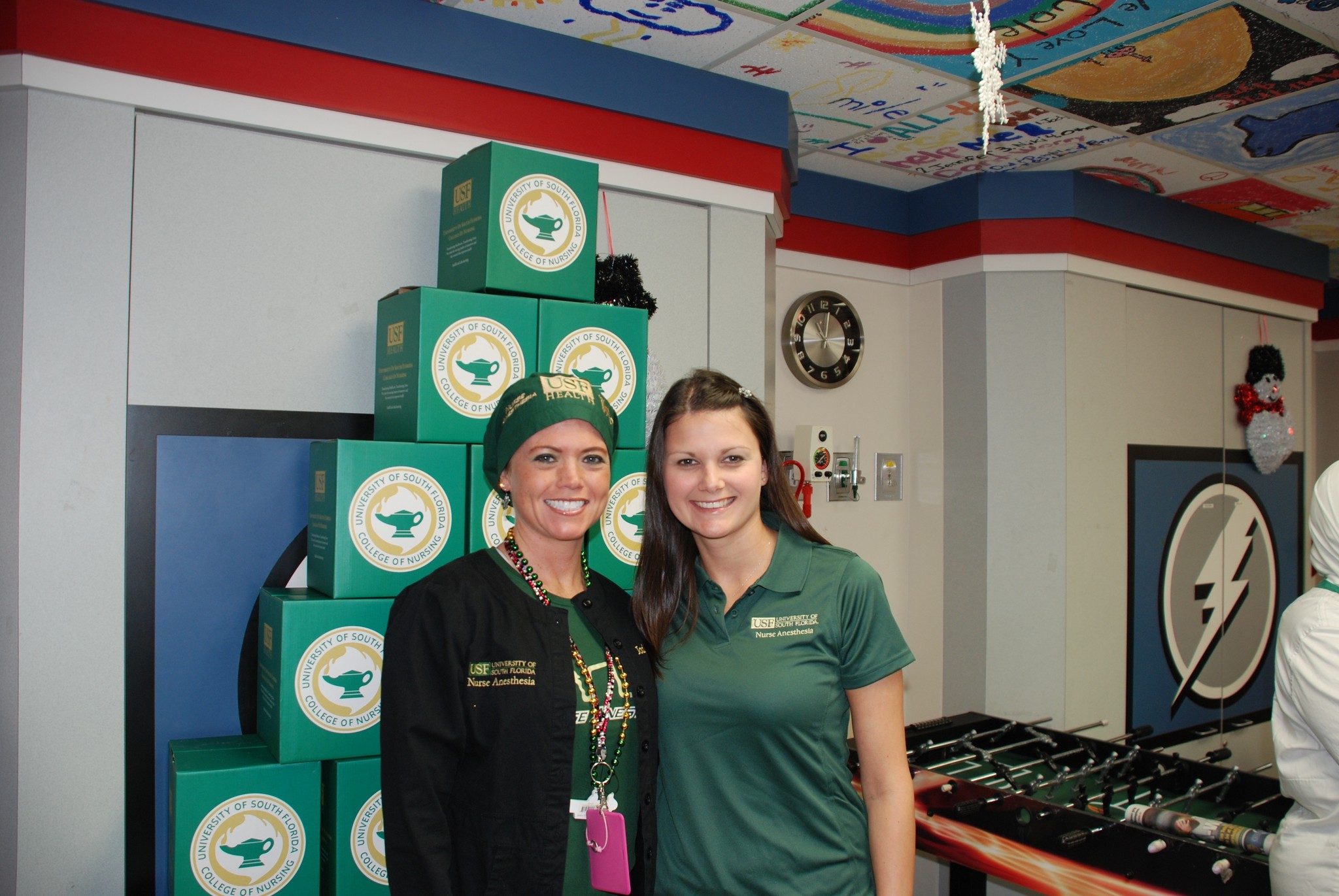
[160,143,649,896]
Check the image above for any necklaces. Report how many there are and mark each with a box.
[503,526,630,791]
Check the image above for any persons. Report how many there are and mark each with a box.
[615,369,917,896]
[1268,460,1337,895]
[911,720,1282,832]
[376,376,656,895]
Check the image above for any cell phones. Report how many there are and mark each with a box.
[585,810,630,895]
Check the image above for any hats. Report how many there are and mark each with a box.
[482,373,619,507]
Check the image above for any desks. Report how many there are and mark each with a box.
[845,708,1295,896]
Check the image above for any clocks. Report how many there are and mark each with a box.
[781,290,866,390]
